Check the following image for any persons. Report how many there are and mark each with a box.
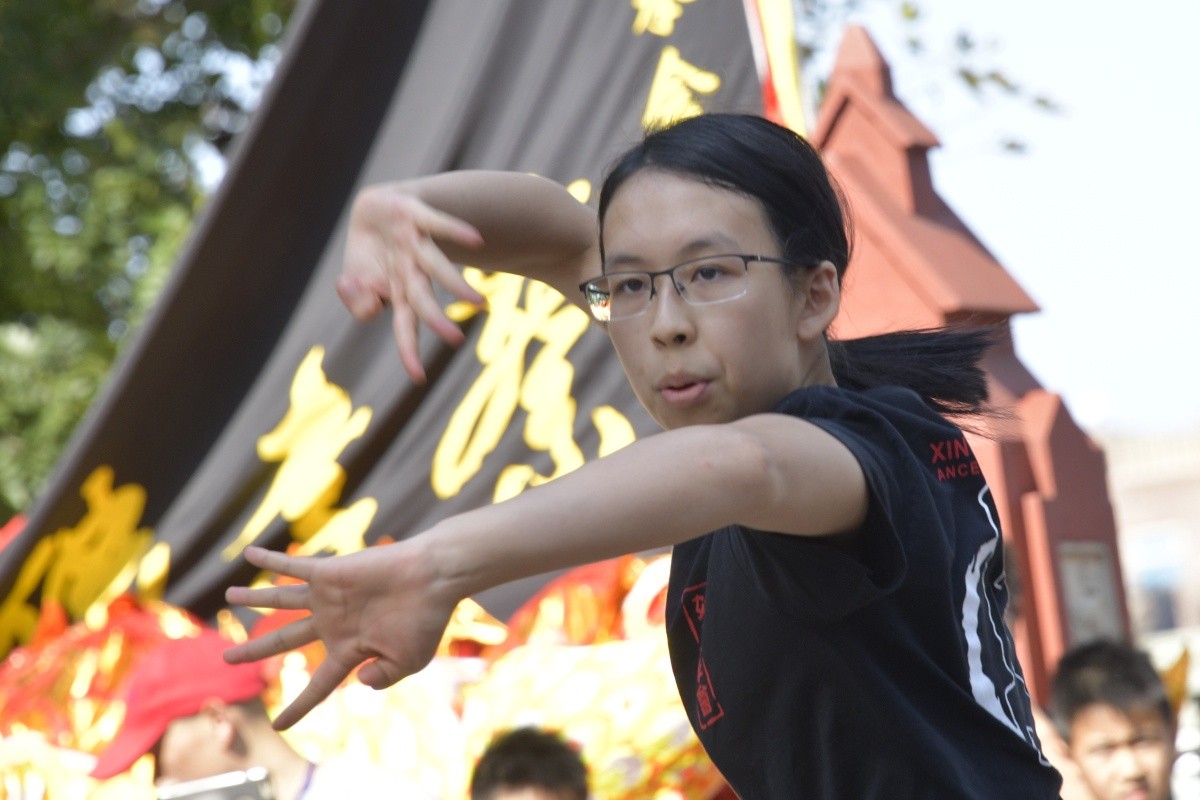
[1049,639,1177,799]
[91,636,333,798]
[469,725,591,800]
[222,112,1064,800]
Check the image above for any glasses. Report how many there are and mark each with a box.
[578,253,796,321]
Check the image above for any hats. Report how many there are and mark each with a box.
[95,628,270,777]
